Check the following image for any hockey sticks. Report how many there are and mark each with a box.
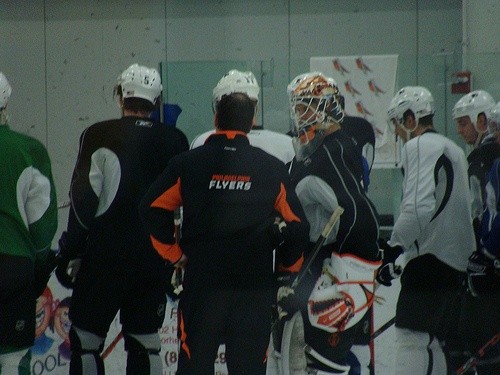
[456,333,499,375]
[174,205,182,288]
[269,206,344,332]
[374,263,402,292]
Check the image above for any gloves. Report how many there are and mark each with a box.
[466,247,500,282]
[55,230,78,289]
[376,243,404,286]
[166,255,184,302]
[276,283,296,323]
[35,259,53,297]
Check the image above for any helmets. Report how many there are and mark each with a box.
[113,63,163,105]
[213,69,260,103]
[387,86,435,120]
[452,89,495,123]
[0,72,12,107]
[489,101,500,131]
[287,71,345,140]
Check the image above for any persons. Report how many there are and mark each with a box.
[146,91,310,375]
[0,73,57,375]
[189,68,500,375]
[54,63,188,375]
